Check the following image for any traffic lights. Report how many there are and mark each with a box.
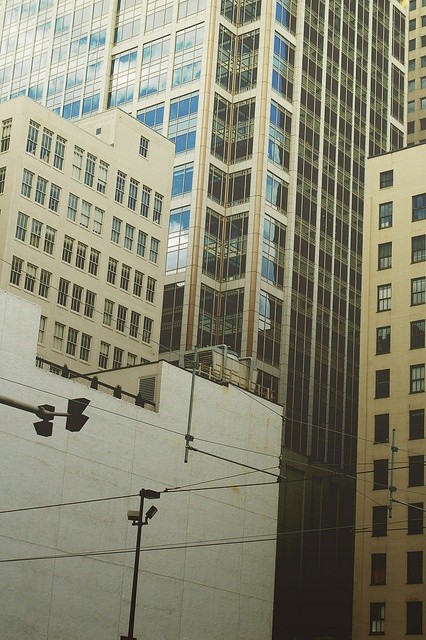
[66,399,89,432]
[34,405,55,437]
